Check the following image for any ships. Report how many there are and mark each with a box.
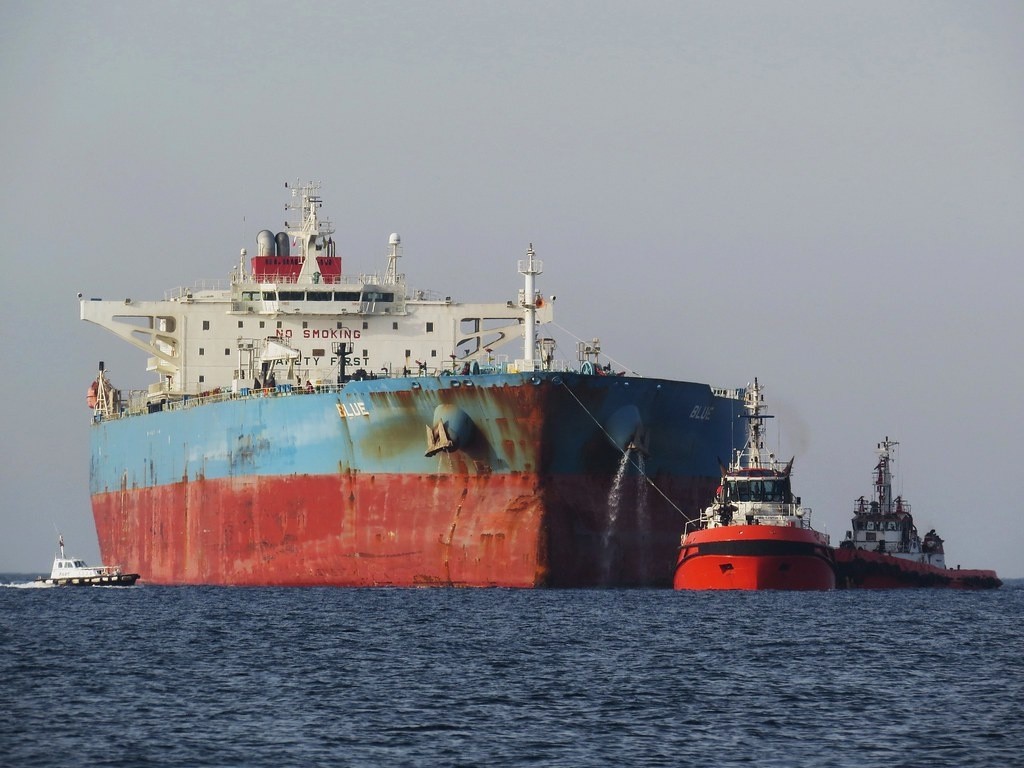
[77,178,750,588]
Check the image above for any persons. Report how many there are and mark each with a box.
[305,380,314,393]
[297,376,301,386]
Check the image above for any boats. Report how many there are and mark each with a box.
[35,535,141,586]
[835,436,1004,589]
[673,377,836,590]
